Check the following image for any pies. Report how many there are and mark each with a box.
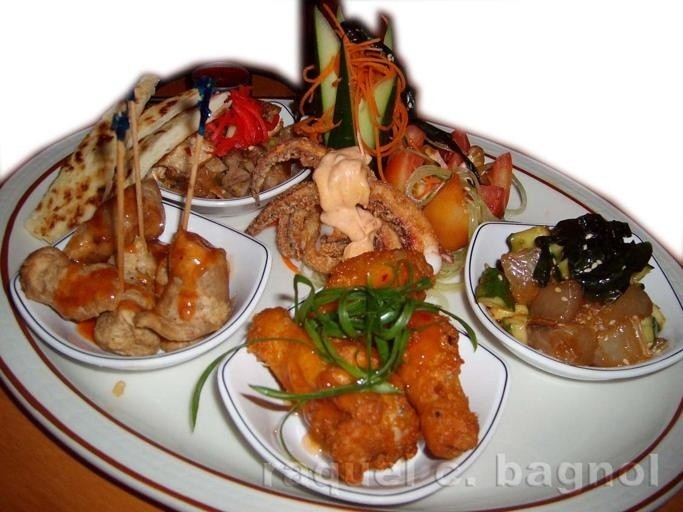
[24,73,159,244]
[126,89,199,153]
[109,91,233,198]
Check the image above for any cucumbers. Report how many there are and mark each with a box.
[301,0,397,180]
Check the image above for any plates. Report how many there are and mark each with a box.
[220,299,512,508]
[147,100,313,216]
[8,193,273,371]
[0,94,683,511]
[464,220,682,384]
